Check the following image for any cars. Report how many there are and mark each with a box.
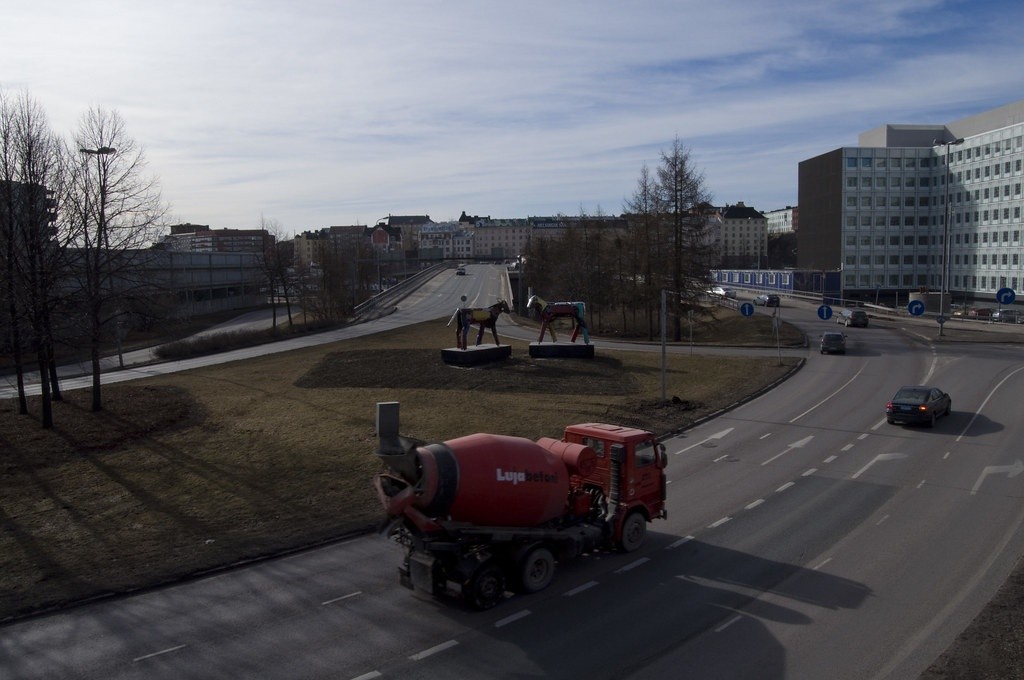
[818,331,849,355]
[992,309,1023,323]
[836,309,872,328]
[455,266,465,275]
[952,306,971,318]
[887,382,954,430]
[752,294,780,307]
[706,286,736,299]
[968,307,998,321]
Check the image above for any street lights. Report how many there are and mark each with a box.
[80,146,127,371]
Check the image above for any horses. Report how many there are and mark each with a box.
[446,300,510,350]
[526,295,590,344]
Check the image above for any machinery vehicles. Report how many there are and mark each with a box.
[368,398,671,617]
